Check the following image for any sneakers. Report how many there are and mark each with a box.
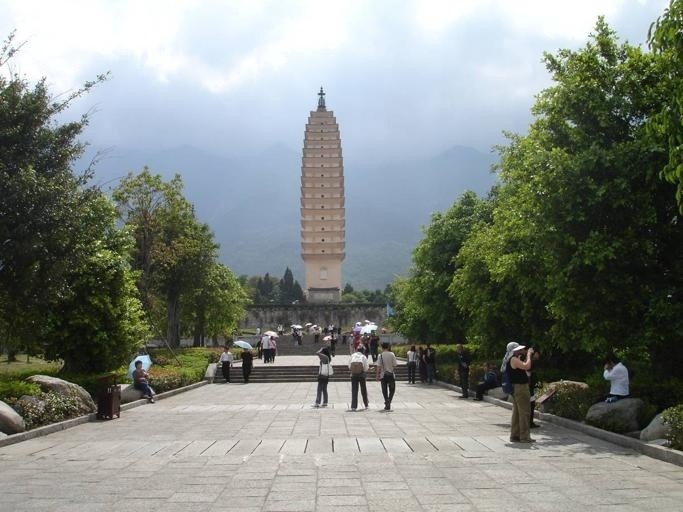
[257,357,274,364]
[459,395,483,401]
[509,422,539,444]
[308,399,392,411]
[408,379,433,385]
[141,394,155,404]
[222,378,248,383]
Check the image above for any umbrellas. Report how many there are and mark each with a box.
[353,319,378,334]
[128,354,153,379]
[233,341,253,350]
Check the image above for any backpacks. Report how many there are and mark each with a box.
[350,362,363,374]
[500,356,515,395]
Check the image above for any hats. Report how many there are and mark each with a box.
[506,341,526,351]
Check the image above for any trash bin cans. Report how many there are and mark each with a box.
[95,374,121,420]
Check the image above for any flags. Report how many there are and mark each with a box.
[387,302,396,318]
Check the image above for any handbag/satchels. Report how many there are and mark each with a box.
[317,374,328,384]
[383,371,394,383]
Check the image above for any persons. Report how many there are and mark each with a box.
[501,340,542,443]
[310,347,331,407]
[376,341,397,409]
[348,345,369,411]
[473,362,498,400]
[241,348,253,383]
[218,346,233,384]
[595,354,631,402]
[291,323,387,363]
[455,344,470,398]
[257,323,285,364]
[132,361,156,403]
[406,343,437,385]
